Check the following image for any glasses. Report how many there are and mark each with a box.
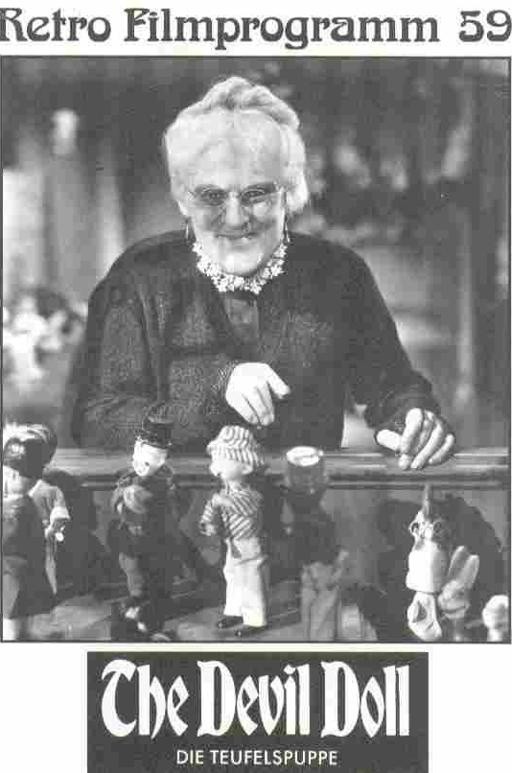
[186,183,288,207]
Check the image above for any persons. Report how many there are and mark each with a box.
[66,73,459,472]
[0,413,511,643]
